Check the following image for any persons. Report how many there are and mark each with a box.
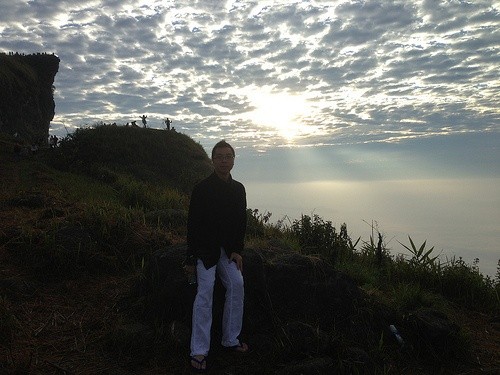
[0,52,177,156]
[182,140,249,371]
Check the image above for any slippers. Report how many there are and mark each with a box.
[225,340,249,354]
[190,355,207,371]
